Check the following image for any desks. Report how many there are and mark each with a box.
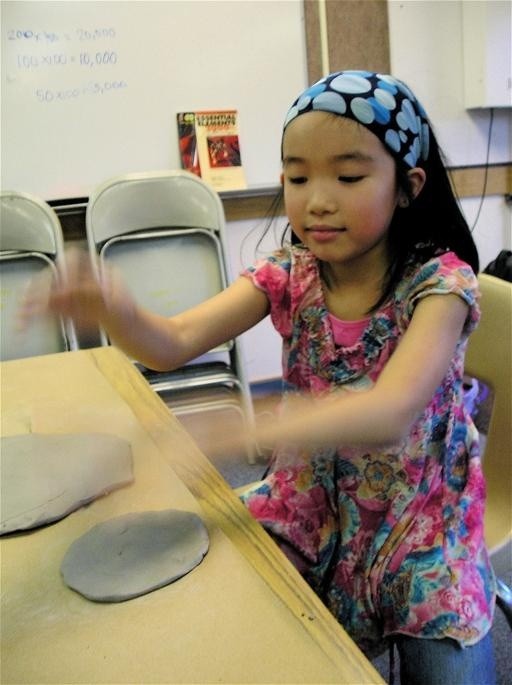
[1,340,393,684]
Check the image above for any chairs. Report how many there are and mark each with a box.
[233,269,512,685]
[0,192,78,359]
[83,172,256,431]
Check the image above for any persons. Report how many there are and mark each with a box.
[21,68,499,685]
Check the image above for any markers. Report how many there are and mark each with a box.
[3,1,308,221]
[47,197,88,206]
